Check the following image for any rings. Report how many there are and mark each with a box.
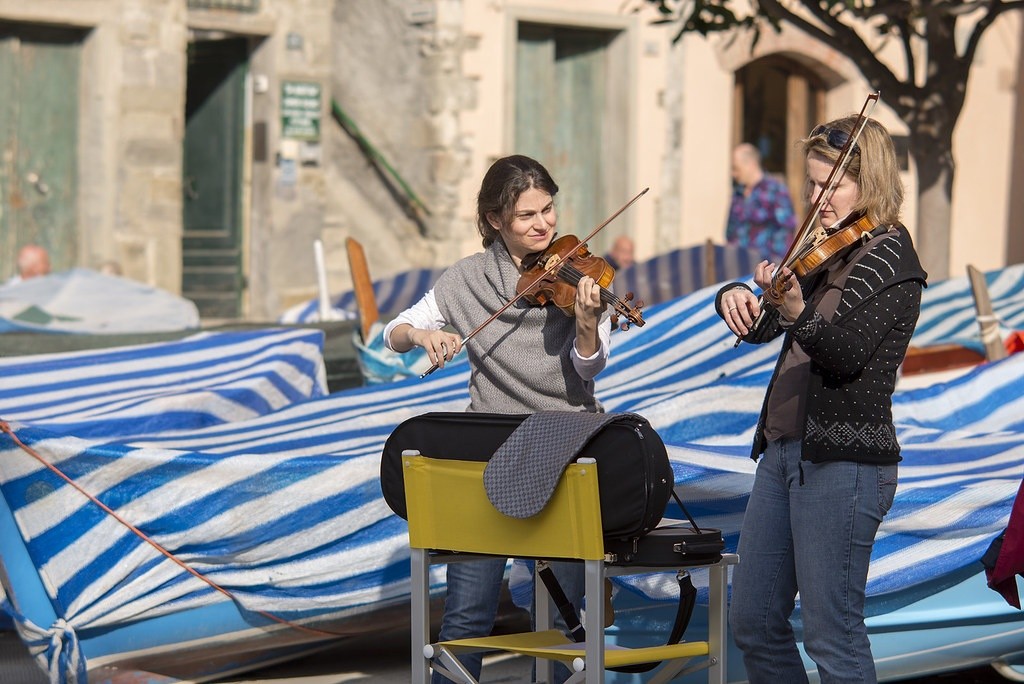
[729,307,737,313]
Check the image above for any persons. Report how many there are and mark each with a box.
[6,243,50,286]
[725,140,796,264]
[716,115,925,684]
[380,153,613,684]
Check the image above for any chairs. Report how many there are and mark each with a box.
[402,450,739,684]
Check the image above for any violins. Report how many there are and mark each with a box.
[516,234,646,331]
[762,212,880,309]
